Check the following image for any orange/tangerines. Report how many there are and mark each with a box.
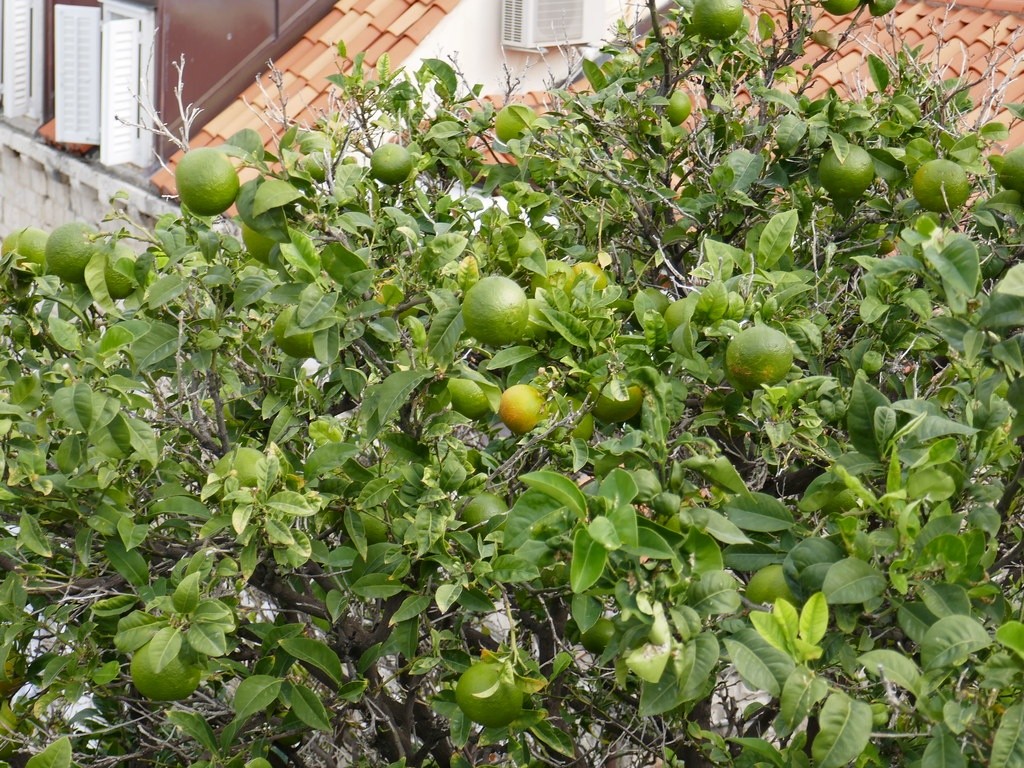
[0,0,1024,727]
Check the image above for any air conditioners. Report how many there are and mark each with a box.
[501,0,590,50]
